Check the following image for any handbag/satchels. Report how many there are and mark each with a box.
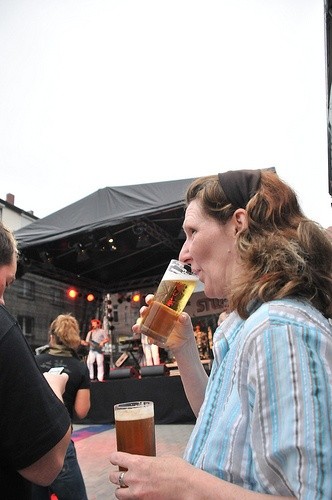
[90,340,105,354]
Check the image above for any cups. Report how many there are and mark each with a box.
[142,259,200,348]
[114,401,156,487]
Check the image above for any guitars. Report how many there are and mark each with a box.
[80,339,105,354]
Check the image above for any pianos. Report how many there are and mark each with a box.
[119,338,144,371]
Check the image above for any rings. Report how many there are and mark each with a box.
[119,472,127,488]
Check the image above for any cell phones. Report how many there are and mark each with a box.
[48,366,64,374]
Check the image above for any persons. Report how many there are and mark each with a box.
[0,226,73,500]
[33,314,89,500]
[107,169,332,500]
[85,320,110,382]
[194,326,206,361]
[137,318,160,366]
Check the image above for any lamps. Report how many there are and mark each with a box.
[65,287,114,331]
[118,292,148,305]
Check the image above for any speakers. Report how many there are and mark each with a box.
[109,365,170,379]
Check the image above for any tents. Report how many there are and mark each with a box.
[11,177,208,297]
